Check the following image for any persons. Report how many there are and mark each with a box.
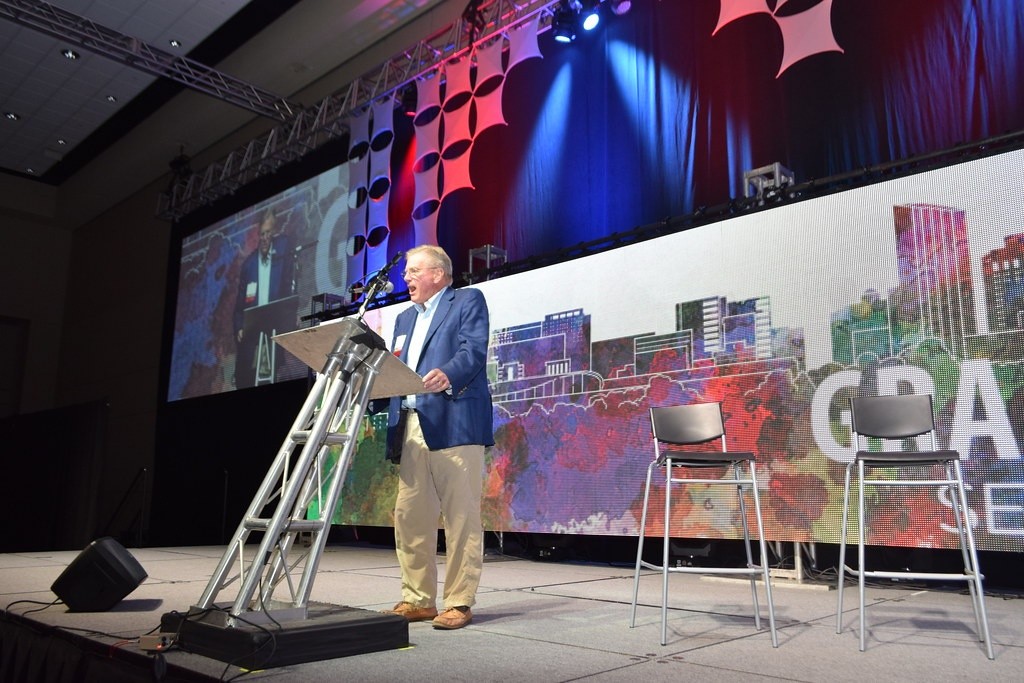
[360,245,496,630]
[234,209,296,402]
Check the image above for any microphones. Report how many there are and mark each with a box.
[349,282,394,293]
[272,249,277,264]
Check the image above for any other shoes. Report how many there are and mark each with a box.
[382,601,438,622]
[432,608,474,630]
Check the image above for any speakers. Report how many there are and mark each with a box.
[51,536,148,613]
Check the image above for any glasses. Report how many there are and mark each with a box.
[259,230,272,240]
[400,266,438,279]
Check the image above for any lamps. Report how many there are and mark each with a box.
[552,0,631,43]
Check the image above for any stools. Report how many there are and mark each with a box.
[837,392,994,661]
[631,403,785,649]
